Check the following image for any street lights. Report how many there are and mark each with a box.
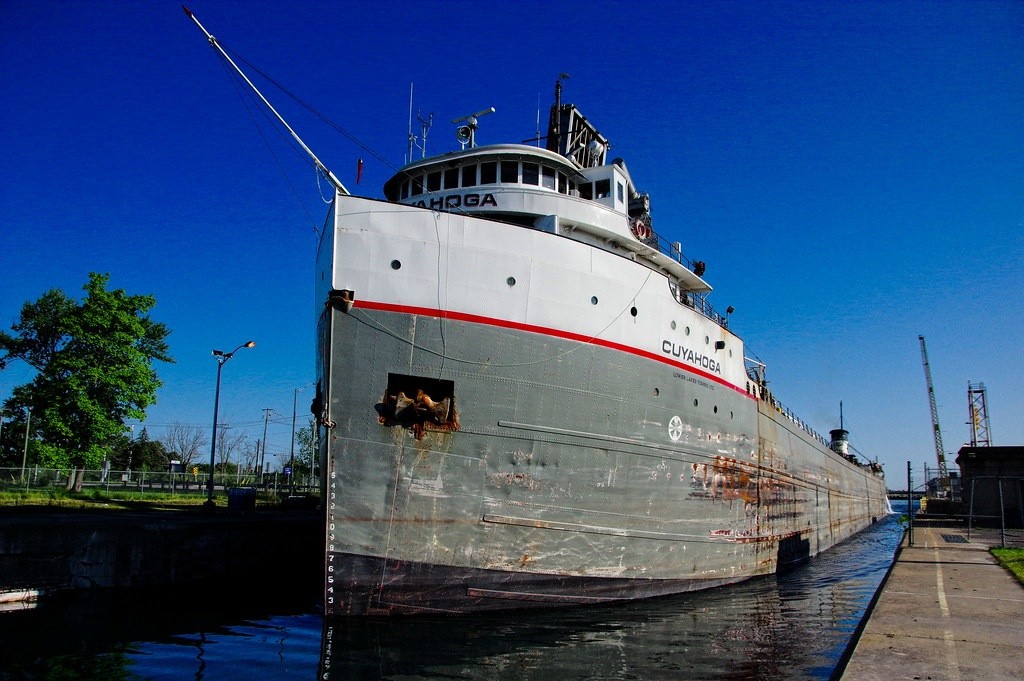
[202,341,256,506]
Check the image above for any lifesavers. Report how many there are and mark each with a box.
[635,219,647,241]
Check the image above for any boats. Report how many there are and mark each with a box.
[310,72,886,617]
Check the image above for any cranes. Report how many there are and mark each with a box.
[919,335,950,498]
